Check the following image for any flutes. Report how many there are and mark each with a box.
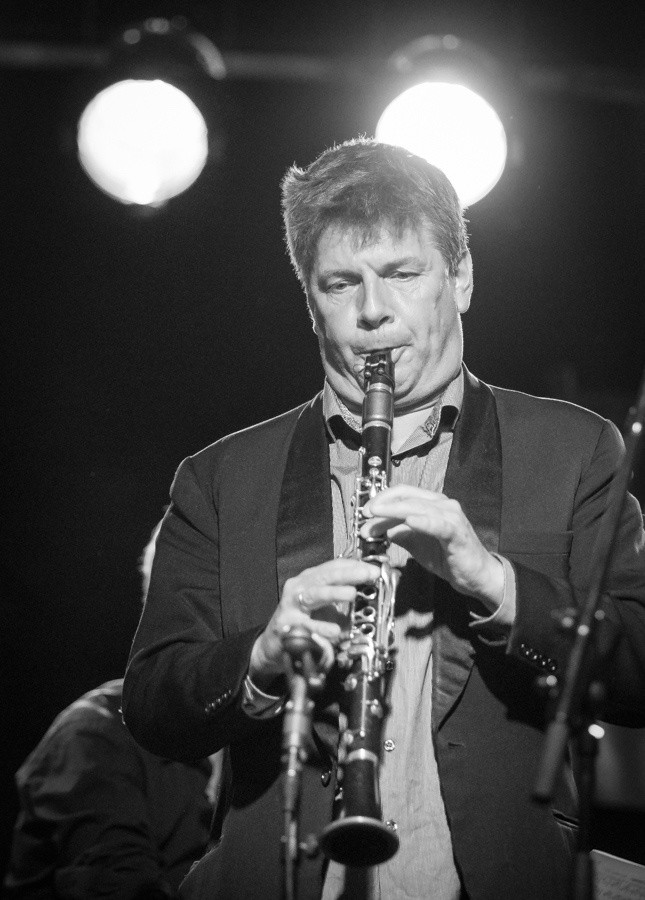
[318,348,401,866]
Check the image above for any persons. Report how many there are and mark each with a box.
[121,137,645,899]
[0,506,224,899]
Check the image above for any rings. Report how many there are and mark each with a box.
[297,590,313,613]
